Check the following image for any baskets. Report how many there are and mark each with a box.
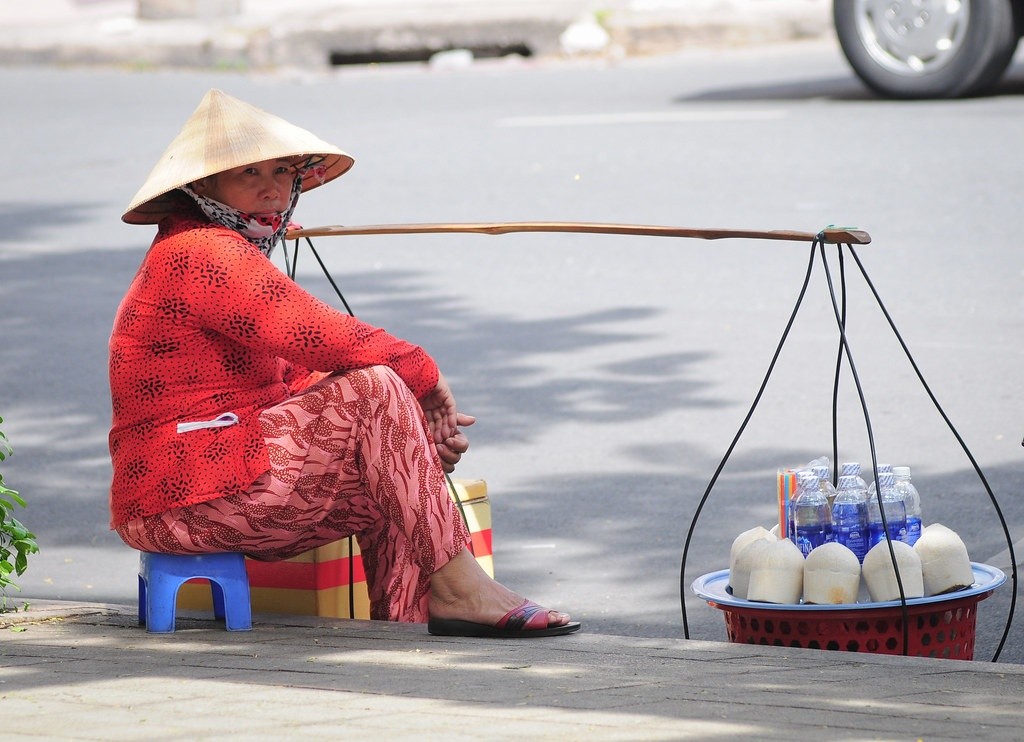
[701,587,997,660]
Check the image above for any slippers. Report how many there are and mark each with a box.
[428,598,582,636]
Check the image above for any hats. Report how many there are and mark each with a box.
[121,87,354,226]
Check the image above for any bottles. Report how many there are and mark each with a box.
[786,460,922,563]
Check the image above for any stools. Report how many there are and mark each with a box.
[138,549,249,634]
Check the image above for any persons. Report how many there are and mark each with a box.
[106,87,581,639]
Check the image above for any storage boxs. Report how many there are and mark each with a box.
[174,482,493,621]
